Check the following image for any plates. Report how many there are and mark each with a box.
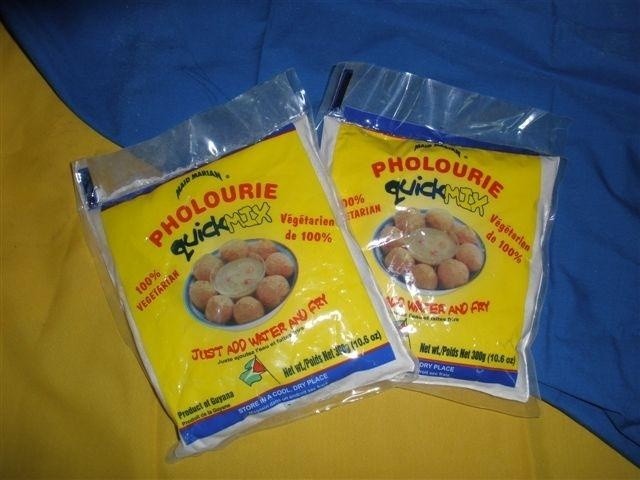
[372,209,487,294]
[183,239,299,329]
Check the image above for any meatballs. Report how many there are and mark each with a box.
[190,239,294,325]
[379,207,484,289]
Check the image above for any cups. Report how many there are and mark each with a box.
[403,221,460,264]
[210,250,265,299]
